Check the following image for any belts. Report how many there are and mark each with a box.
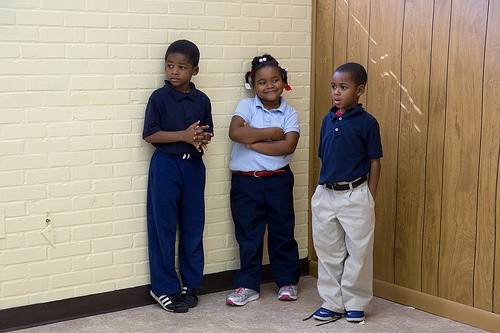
[326,176,367,191]
[233,170,286,177]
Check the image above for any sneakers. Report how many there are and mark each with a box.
[150,286,199,313]
[226,288,260,306]
[278,285,298,300]
[313,307,365,322]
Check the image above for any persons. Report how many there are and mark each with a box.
[142,40,215,313]
[226,54,300,305]
[311,62,384,321]
